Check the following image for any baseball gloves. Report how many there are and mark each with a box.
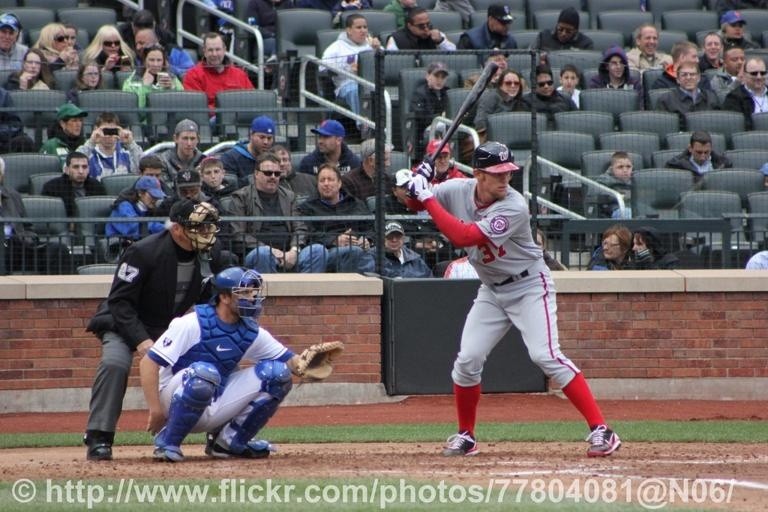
[292,342,346,383]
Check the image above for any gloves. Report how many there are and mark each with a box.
[290,339,347,385]
[407,157,437,199]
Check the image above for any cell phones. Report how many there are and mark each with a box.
[120,55,130,67]
[102,128,118,136]
[156,72,168,84]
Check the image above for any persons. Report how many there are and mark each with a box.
[78,195,221,463]
[139,265,343,464]
[1,0,767,273]
[396,136,622,456]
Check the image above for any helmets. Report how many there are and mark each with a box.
[171,196,219,251]
[208,265,267,321]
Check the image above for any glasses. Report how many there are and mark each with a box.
[501,18,768,89]
[602,240,622,247]
[416,23,435,31]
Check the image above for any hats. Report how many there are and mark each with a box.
[427,62,450,77]
[426,137,452,157]
[721,9,747,24]
[486,2,515,23]
[471,139,521,174]
[1,9,415,237]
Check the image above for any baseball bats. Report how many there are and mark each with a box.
[405,62,499,201]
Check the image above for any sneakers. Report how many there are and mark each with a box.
[204,439,271,459]
[85,443,115,461]
[439,432,480,459]
[152,444,187,464]
[584,425,622,459]
